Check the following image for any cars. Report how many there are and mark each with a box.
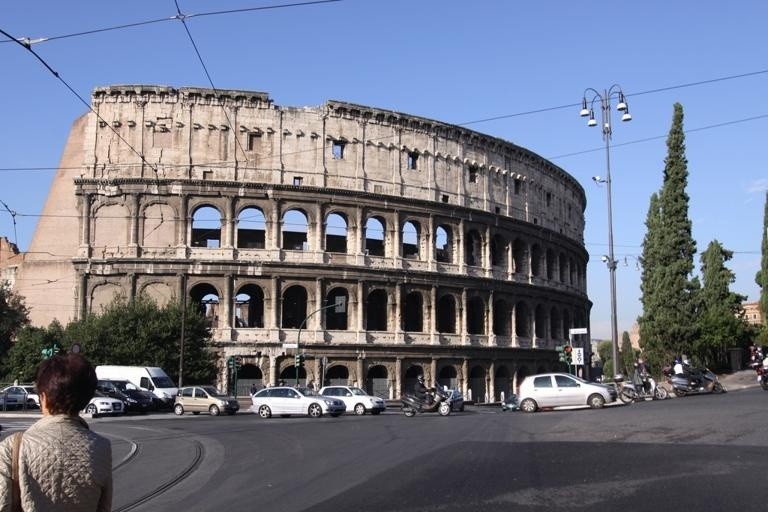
[248,385,346,419]
[0,383,40,410]
[445,388,464,412]
[80,389,125,418]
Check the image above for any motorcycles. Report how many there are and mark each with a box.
[613,371,669,404]
[662,366,723,397]
[750,361,767,390]
[400,387,451,417]
[747,345,764,362]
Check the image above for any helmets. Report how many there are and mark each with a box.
[418,375,425,382]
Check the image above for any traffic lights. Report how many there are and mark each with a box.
[42,344,61,360]
[294,354,305,368]
[555,343,573,362]
[228,355,242,371]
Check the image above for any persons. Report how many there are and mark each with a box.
[762,353,768,372]
[250,383,257,399]
[673,358,693,390]
[638,357,650,395]
[682,359,705,385]
[294,381,300,387]
[413,374,433,405]
[307,380,314,395]
[634,361,647,394]
[0,352,114,511]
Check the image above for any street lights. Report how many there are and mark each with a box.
[176,272,188,388]
[624,254,644,270]
[580,83,632,397]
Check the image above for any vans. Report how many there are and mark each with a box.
[93,364,180,410]
[95,379,154,415]
[318,384,386,416]
[515,372,618,411]
[172,384,241,417]
